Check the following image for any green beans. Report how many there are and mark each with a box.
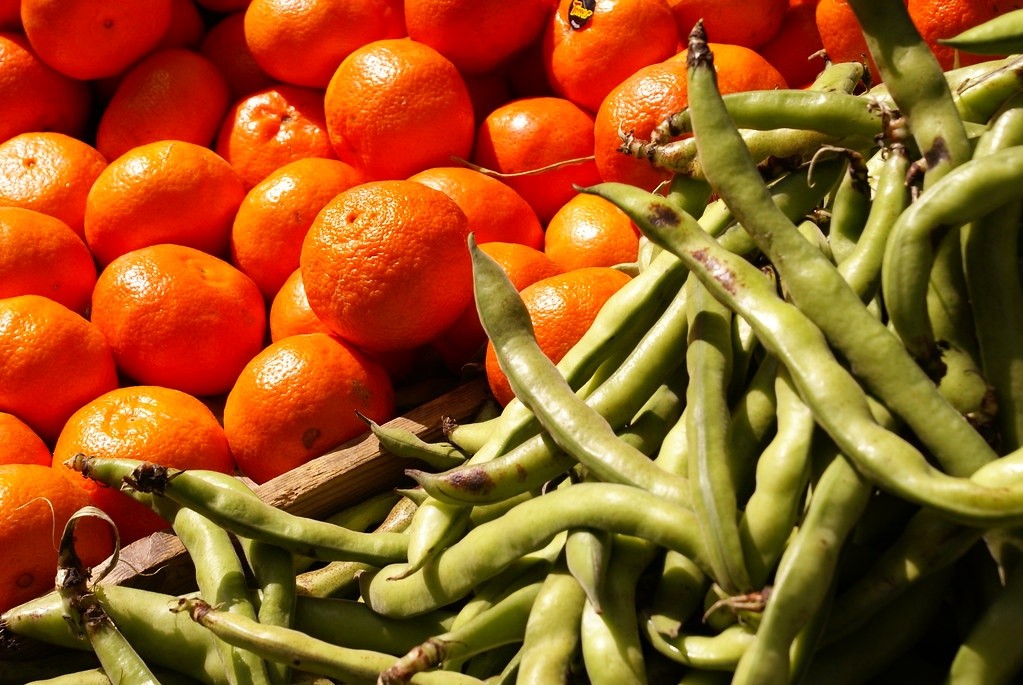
[0,414,521,685]
[346,0,1023,685]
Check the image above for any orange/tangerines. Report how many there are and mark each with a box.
[0,0,1021,587]
